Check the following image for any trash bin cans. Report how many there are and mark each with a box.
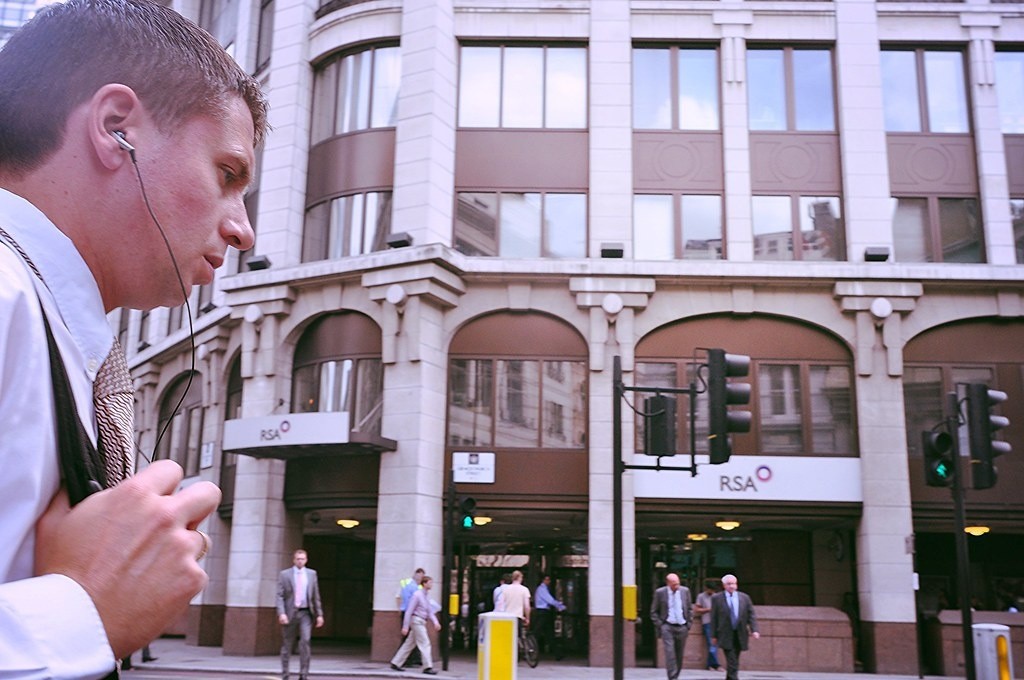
[971,623,1014,680]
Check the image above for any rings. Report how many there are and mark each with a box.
[192,528,208,562]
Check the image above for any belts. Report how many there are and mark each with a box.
[298,607,309,611]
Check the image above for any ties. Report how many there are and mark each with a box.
[672,592,682,626]
[0,226,135,494]
[295,572,303,607]
[729,593,736,625]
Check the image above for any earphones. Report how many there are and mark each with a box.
[110,131,136,152]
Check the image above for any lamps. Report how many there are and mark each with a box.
[243,254,270,271]
[136,340,151,354]
[386,232,414,248]
[199,301,217,315]
[600,242,625,258]
[864,247,891,261]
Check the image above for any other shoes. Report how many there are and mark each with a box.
[143,656,157,662]
[391,664,405,671]
[708,665,725,671]
[423,669,436,674]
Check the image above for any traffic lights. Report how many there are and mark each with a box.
[964,382,1012,493]
[708,350,753,466]
[917,429,951,489]
[456,496,474,532]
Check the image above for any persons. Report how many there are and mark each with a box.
[0,0,274,680]
[275,550,324,680]
[651,573,694,680]
[840,591,864,667]
[934,585,1024,613]
[493,571,531,662]
[389,568,442,675]
[694,574,760,680]
[534,574,567,662]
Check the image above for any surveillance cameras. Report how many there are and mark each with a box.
[310,514,321,523]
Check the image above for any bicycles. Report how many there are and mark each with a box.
[517,626,540,669]
